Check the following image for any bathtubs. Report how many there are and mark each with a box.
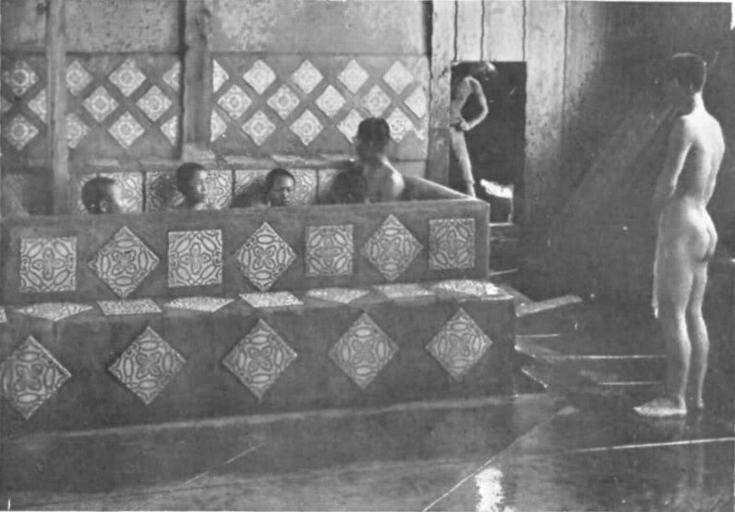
[0,158,492,290]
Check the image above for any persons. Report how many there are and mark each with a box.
[167,161,223,210]
[356,116,410,201]
[261,167,298,206]
[450,61,490,198]
[630,50,727,419]
[79,176,130,216]
